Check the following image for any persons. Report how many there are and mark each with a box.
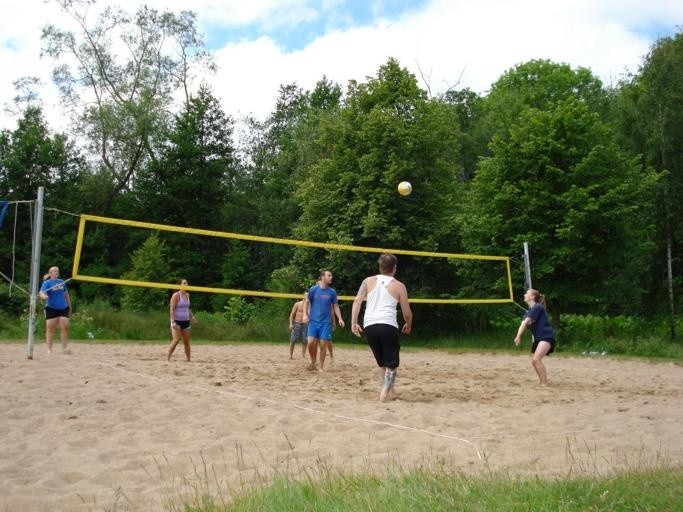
[39,266,73,354]
[350,254,411,403]
[288,268,345,374]
[166,279,198,362]
[513,288,556,386]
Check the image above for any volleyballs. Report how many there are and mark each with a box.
[398,181,412,196]
